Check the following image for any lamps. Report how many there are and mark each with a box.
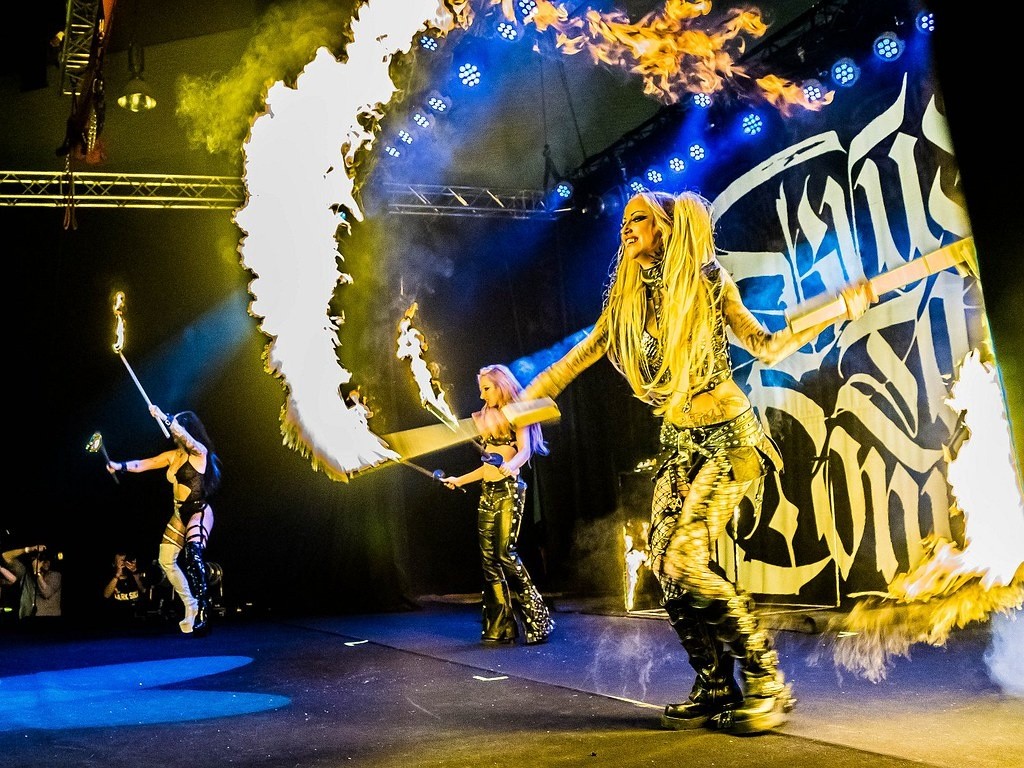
[116,40,157,113]
[384,0,937,219]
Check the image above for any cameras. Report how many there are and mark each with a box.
[40,549,46,564]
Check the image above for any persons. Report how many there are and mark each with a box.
[436,364,556,646]
[2,543,61,619]
[106,405,223,634]
[476,192,879,736]
[103,549,149,626]
[0,565,18,584]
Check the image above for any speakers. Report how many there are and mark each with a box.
[932,0,1024,497]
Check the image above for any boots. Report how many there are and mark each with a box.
[694,586,794,734]
[183,541,219,634]
[159,544,200,633]
[659,593,744,731]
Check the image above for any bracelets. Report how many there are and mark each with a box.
[114,576,119,579]
[35,572,42,578]
[121,462,127,473]
[164,413,175,427]
[25,547,29,553]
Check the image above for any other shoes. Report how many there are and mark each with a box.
[483,636,517,645]
